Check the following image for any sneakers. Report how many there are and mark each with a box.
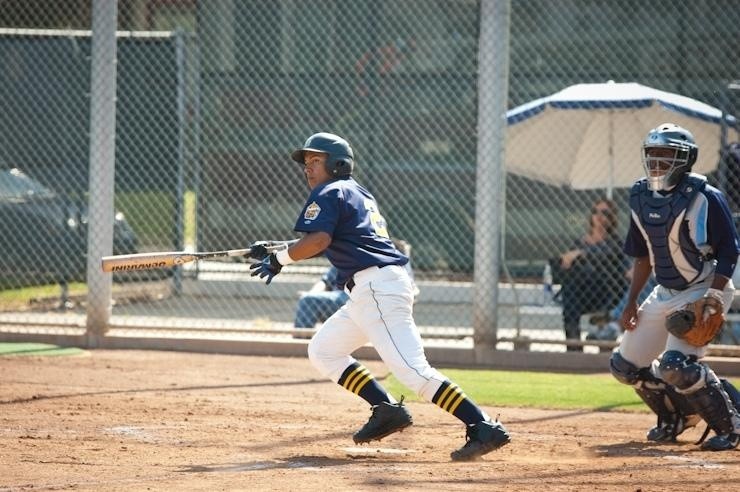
[698,430,740,451]
[450,419,512,461]
[351,400,414,446]
[645,412,703,440]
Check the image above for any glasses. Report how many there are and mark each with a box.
[590,206,609,216]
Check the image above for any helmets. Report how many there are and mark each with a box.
[291,131,355,181]
[640,122,699,193]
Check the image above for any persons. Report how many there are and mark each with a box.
[244,131,511,461]
[294,236,416,338]
[609,124,740,451]
[550,200,633,353]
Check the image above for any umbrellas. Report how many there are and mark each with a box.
[505,80,740,200]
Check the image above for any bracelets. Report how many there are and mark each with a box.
[276,248,295,268]
[704,288,725,304]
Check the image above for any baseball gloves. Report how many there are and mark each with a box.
[663,288,725,346]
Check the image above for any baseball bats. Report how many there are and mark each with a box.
[99,246,289,273]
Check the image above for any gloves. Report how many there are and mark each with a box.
[248,251,284,285]
[243,243,269,261]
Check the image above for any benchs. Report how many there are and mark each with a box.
[517,299,740,353]
